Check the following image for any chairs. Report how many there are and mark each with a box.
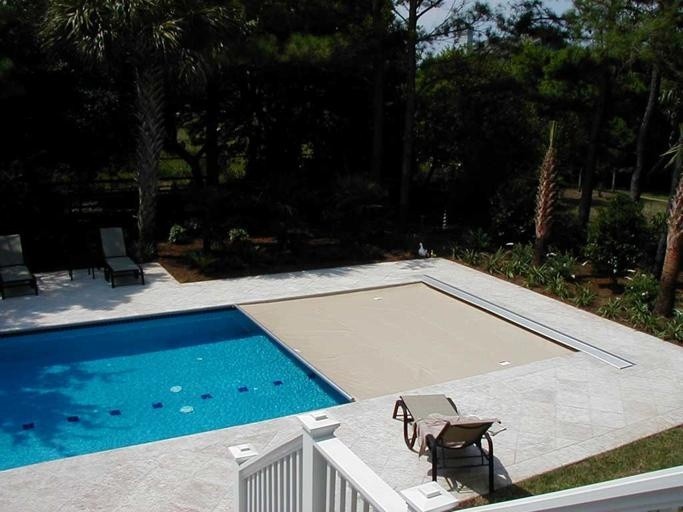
[0,234,38,299]
[97,226,144,289]
[392,391,496,493]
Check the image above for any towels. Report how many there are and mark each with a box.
[414,412,502,459]
